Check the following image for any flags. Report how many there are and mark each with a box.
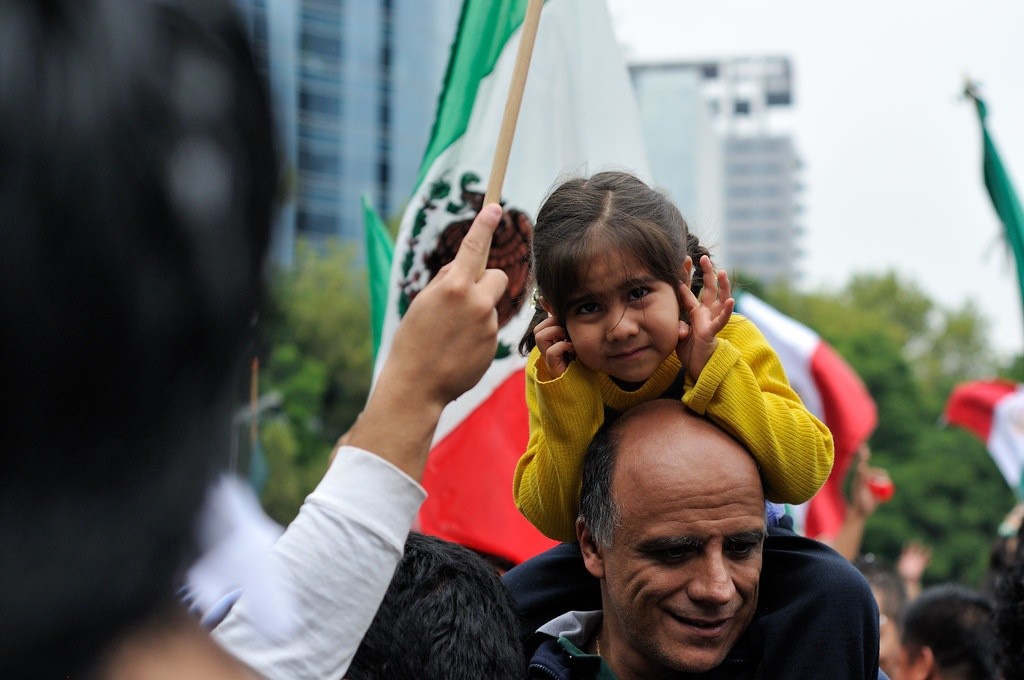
[362,2,878,580]
[976,99,1023,299]
[945,381,1024,505]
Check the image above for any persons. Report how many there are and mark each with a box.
[1,2,285,680]
[499,172,879,680]
[832,465,1024,680]
[183,201,510,680]
[525,398,769,680]
